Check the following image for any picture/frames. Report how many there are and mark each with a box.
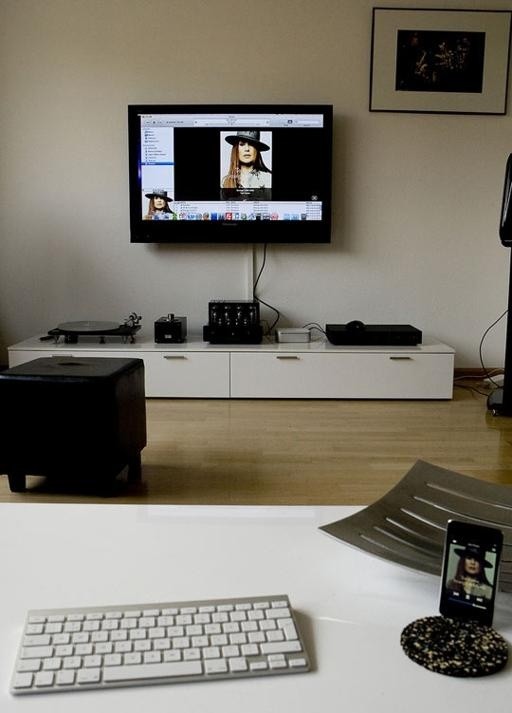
[368,7,512,115]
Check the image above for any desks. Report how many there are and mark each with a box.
[0,501,511,712]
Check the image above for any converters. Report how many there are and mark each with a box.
[481,371,506,390]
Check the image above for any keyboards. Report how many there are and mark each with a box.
[10,594,312,698]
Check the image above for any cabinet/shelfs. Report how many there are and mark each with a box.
[7,331,456,400]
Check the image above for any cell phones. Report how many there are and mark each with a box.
[438,520,504,627]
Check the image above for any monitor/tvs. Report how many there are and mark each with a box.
[127,104,333,244]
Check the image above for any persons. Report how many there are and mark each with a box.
[220,130,273,189]
[447,542,493,600]
[144,189,177,221]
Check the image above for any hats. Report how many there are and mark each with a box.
[225,131,270,151]
[145,190,174,201]
[454,545,493,568]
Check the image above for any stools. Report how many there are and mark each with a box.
[1,356,147,494]
